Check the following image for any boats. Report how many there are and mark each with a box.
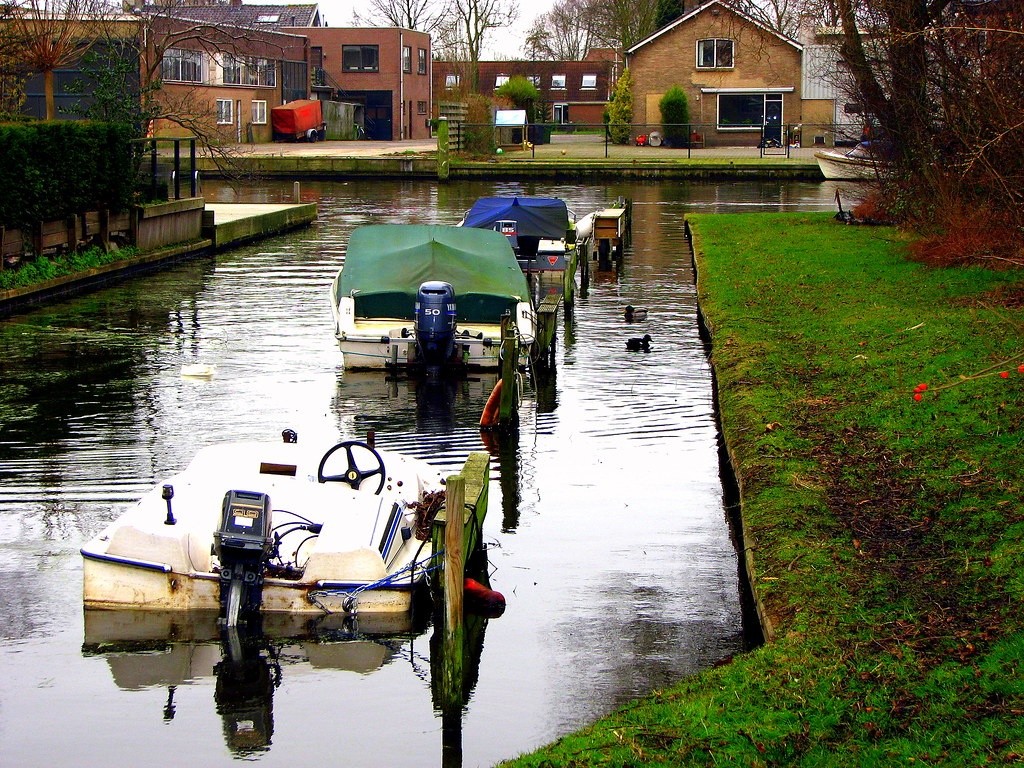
[329,197,582,381]
[814,141,905,181]
[81,427,452,654]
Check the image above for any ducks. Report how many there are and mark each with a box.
[624,333,655,345]
[622,305,648,317]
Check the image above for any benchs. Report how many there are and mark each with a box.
[686,131,706,148]
